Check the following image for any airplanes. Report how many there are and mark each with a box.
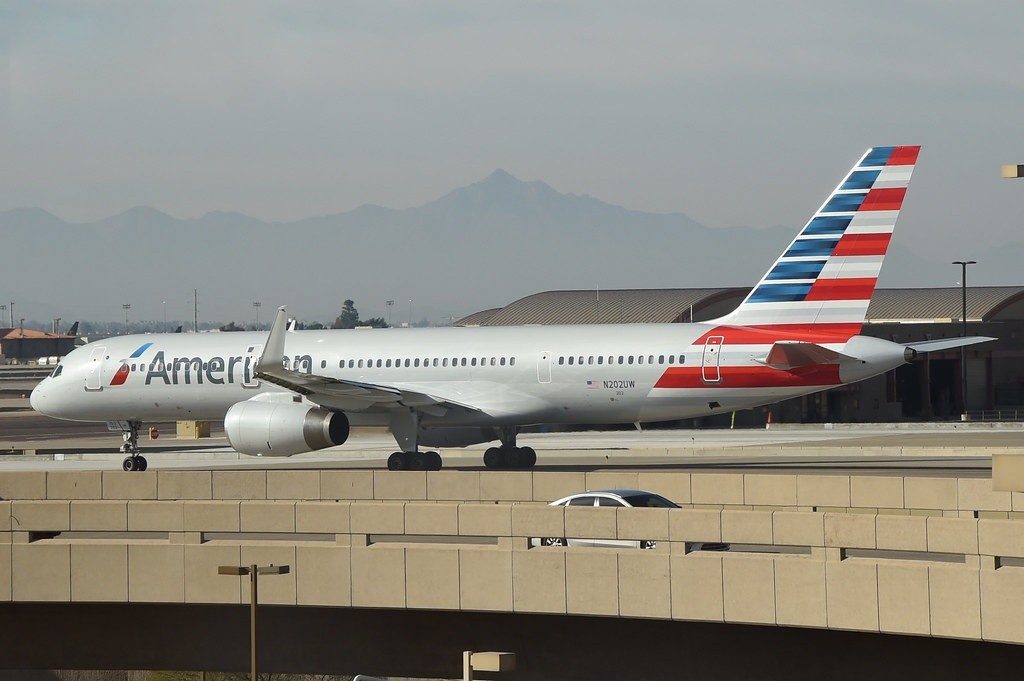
[29,145,1000,473]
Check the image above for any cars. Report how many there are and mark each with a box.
[531,490,728,556]
